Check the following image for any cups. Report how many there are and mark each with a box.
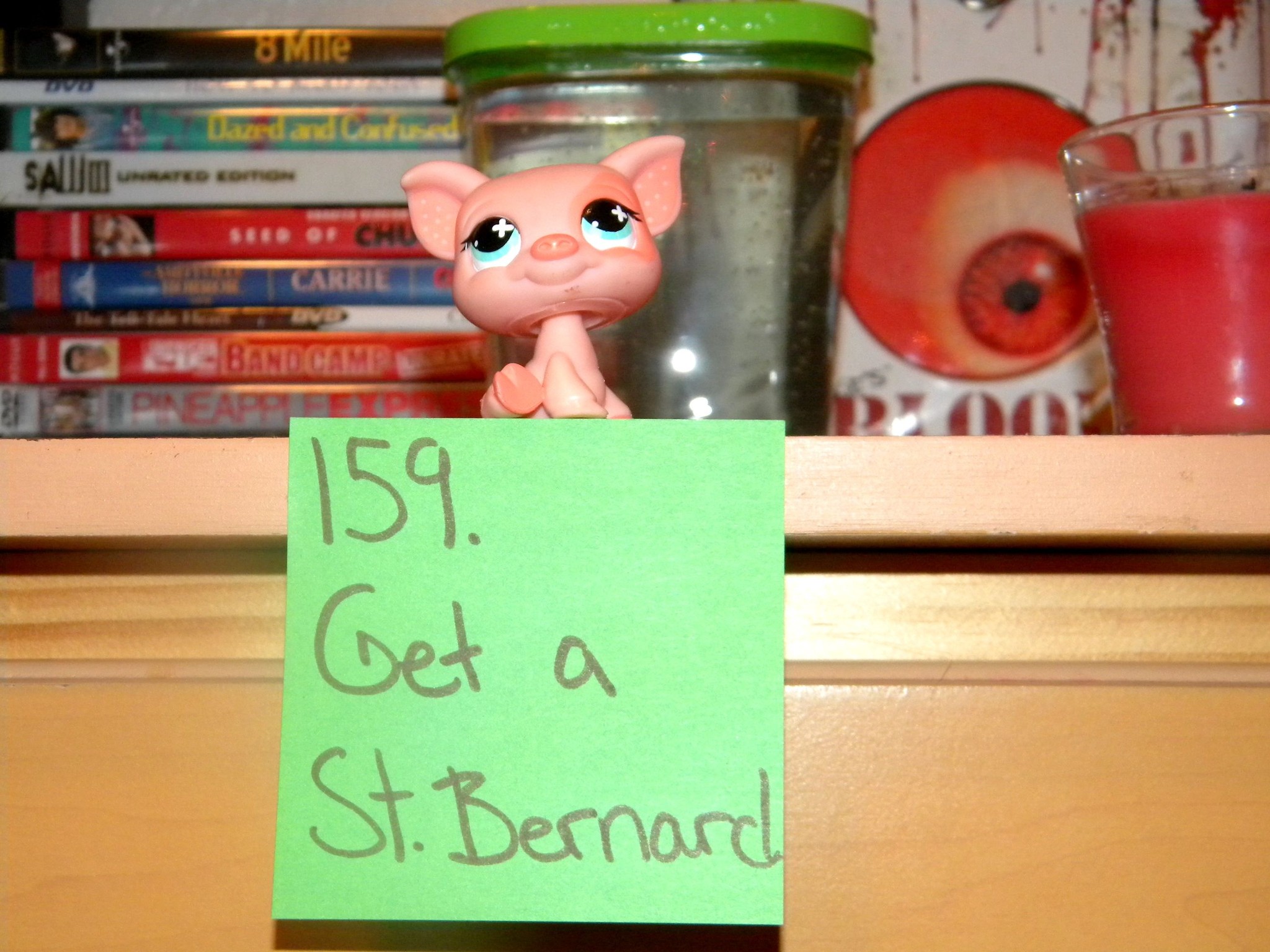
[441,4,878,437]
[1058,99,1270,434]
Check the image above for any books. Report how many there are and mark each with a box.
[0,0,543,436]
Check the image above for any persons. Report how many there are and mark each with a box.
[63,342,119,378]
[30,110,114,152]
